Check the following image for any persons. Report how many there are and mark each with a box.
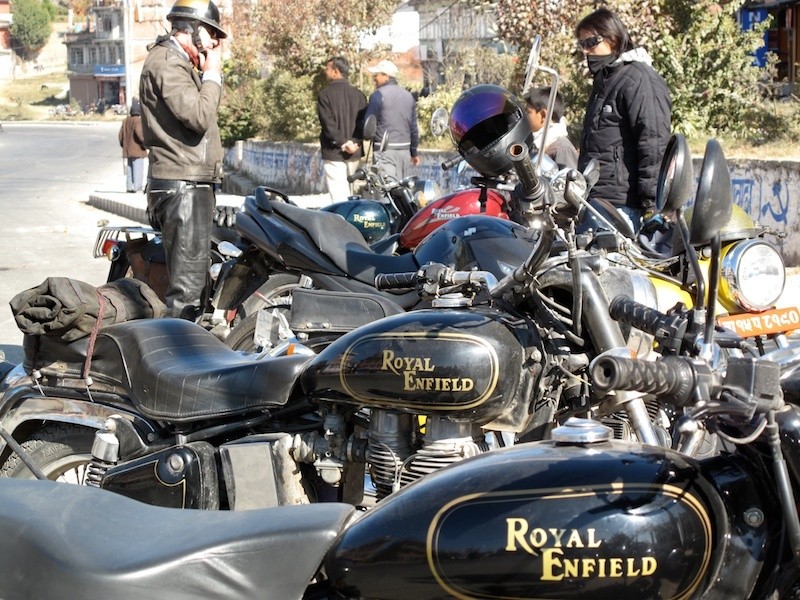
[358,60,420,201]
[142,0,229,326]
[119,94,152,194]
[317,55,371,206]
[523,86,581,177]
[575,10,675,240]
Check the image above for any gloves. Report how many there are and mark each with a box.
[213,206,239,227]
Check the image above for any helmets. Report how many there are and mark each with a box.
[448,84,534,179]
[166,0,227,59]
[638,216,691,259]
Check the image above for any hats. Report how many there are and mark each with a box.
[130,104,142,115]
[368,60,397,77]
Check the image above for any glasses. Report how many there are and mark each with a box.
[579,34,603,49]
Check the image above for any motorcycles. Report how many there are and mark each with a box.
[1,35,800,598]
